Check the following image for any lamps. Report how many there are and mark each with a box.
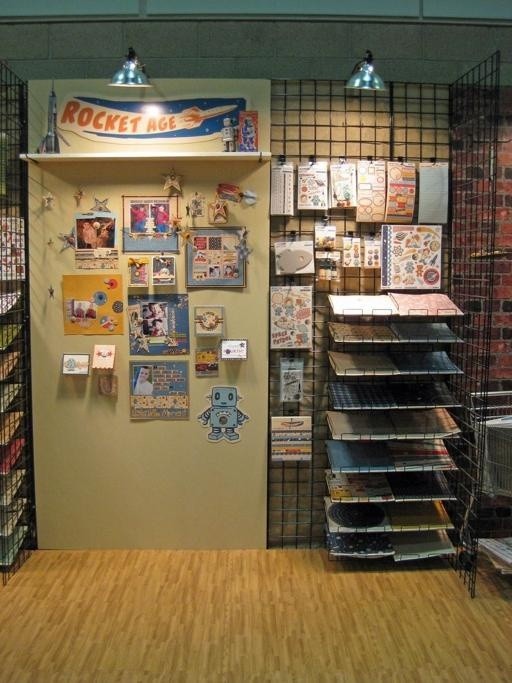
[341,52,387,91]
[109,48,152,88]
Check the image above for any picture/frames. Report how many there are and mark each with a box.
[219,338,248,362]
[194,304,225,338]
[60,353,90,375]
[184,225,248,289]
[128,359,191,420]
[193,345,220,378]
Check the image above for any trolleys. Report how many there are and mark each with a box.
[458,390,512,572]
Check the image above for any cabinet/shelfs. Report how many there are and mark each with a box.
[1,266,34,578]
[325,278,456,563]
